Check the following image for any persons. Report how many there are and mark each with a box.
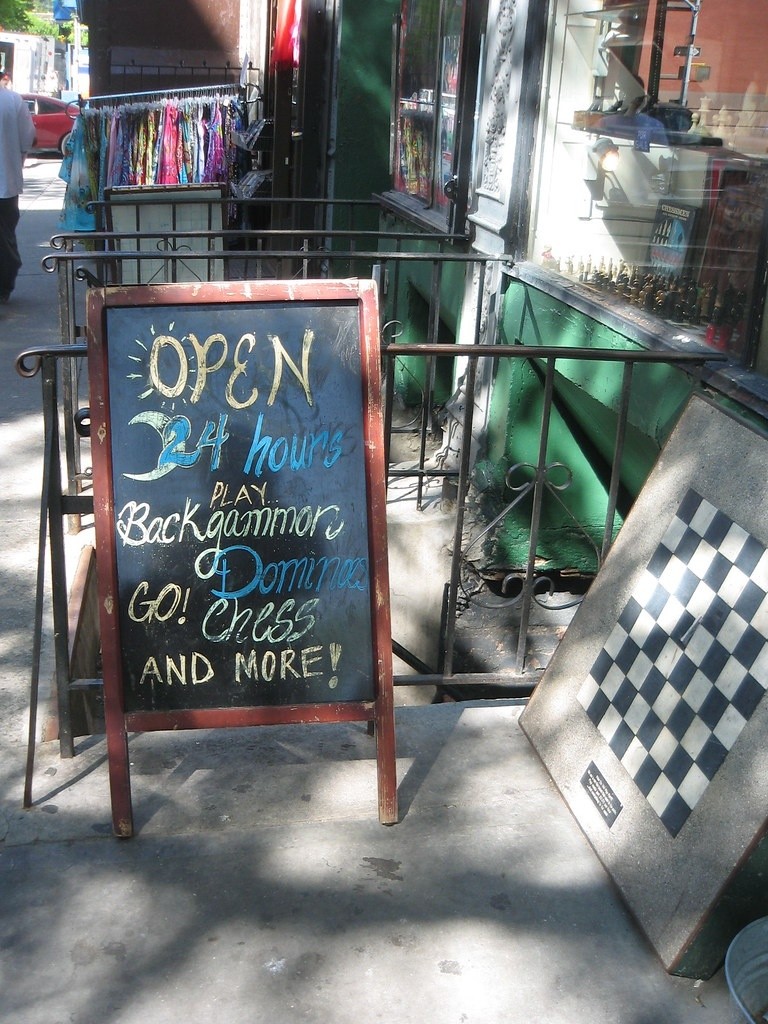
[0,70,35,301]
[441,118,448,151]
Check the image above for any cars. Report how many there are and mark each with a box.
[18,94,79,156]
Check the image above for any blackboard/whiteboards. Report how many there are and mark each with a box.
[83,277,397,725]
